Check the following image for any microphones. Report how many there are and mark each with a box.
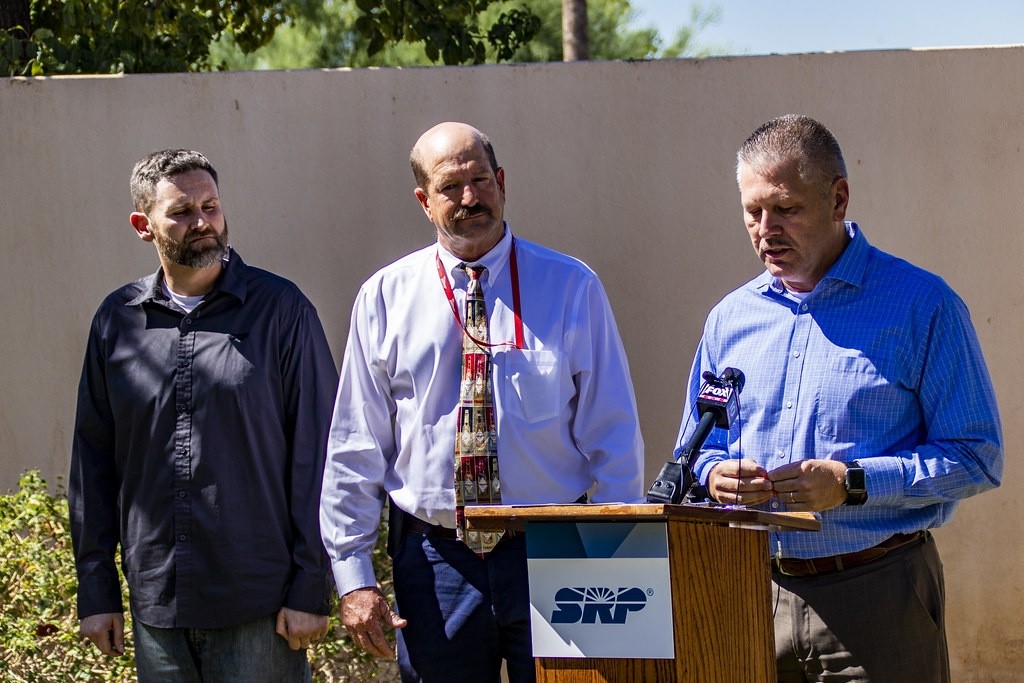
[703,371,720,382]
[725,368,734,380]
[645,366,745,505]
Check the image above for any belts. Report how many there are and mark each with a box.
[770,529,929,577]
[401,510,524,542]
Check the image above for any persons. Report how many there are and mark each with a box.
[67,148,342,683]
[318,121,645,683]
[672,115,1003,683]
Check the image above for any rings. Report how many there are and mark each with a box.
[791,492,795,503]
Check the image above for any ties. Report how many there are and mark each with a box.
[454,262,506,560]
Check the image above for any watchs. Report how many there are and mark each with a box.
[842,462,866,506]
